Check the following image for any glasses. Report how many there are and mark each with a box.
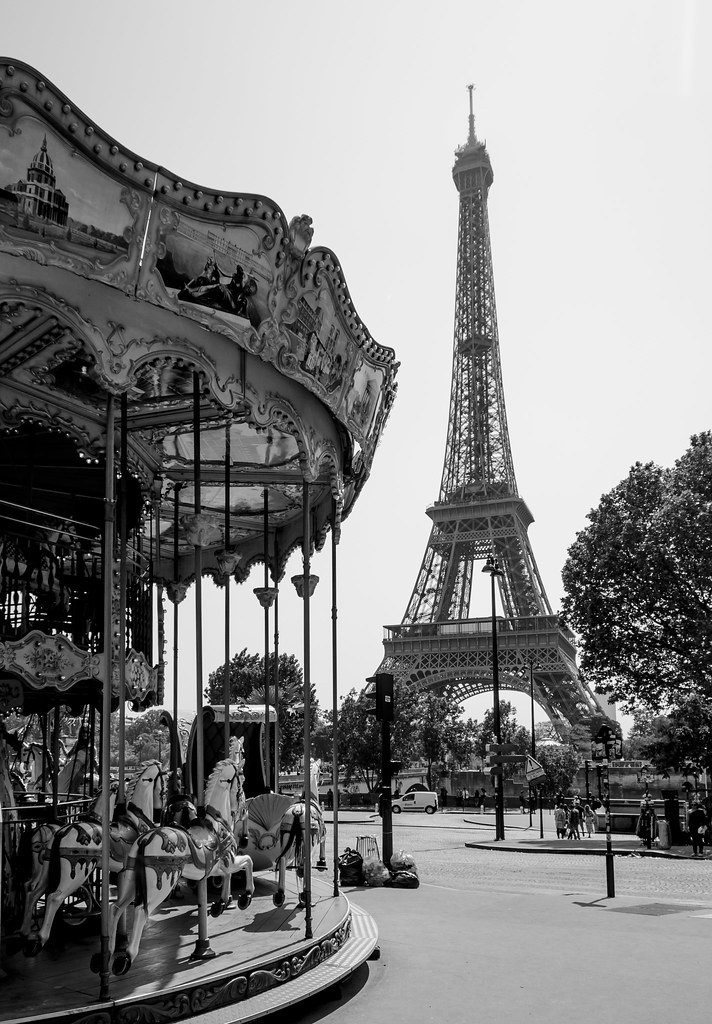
[83,731,89,734]
[692,804,696,806]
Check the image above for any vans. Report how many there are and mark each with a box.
[392,791,438,815]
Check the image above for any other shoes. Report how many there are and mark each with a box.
[692,853,697,856]
[699,854,703,856]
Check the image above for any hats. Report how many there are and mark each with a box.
[569,805,575,807]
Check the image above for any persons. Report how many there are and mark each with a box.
[57,723,101,774]
[298,786,712,857]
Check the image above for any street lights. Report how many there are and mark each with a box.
[482,554,505,841]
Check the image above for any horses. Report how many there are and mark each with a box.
[6,760,167,958]
[88,757,254,976]
[273,757,325,907]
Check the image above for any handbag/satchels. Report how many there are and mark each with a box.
[590,817,595,823]
[560,828,566,833]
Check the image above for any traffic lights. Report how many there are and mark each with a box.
[365,673,394,720]
